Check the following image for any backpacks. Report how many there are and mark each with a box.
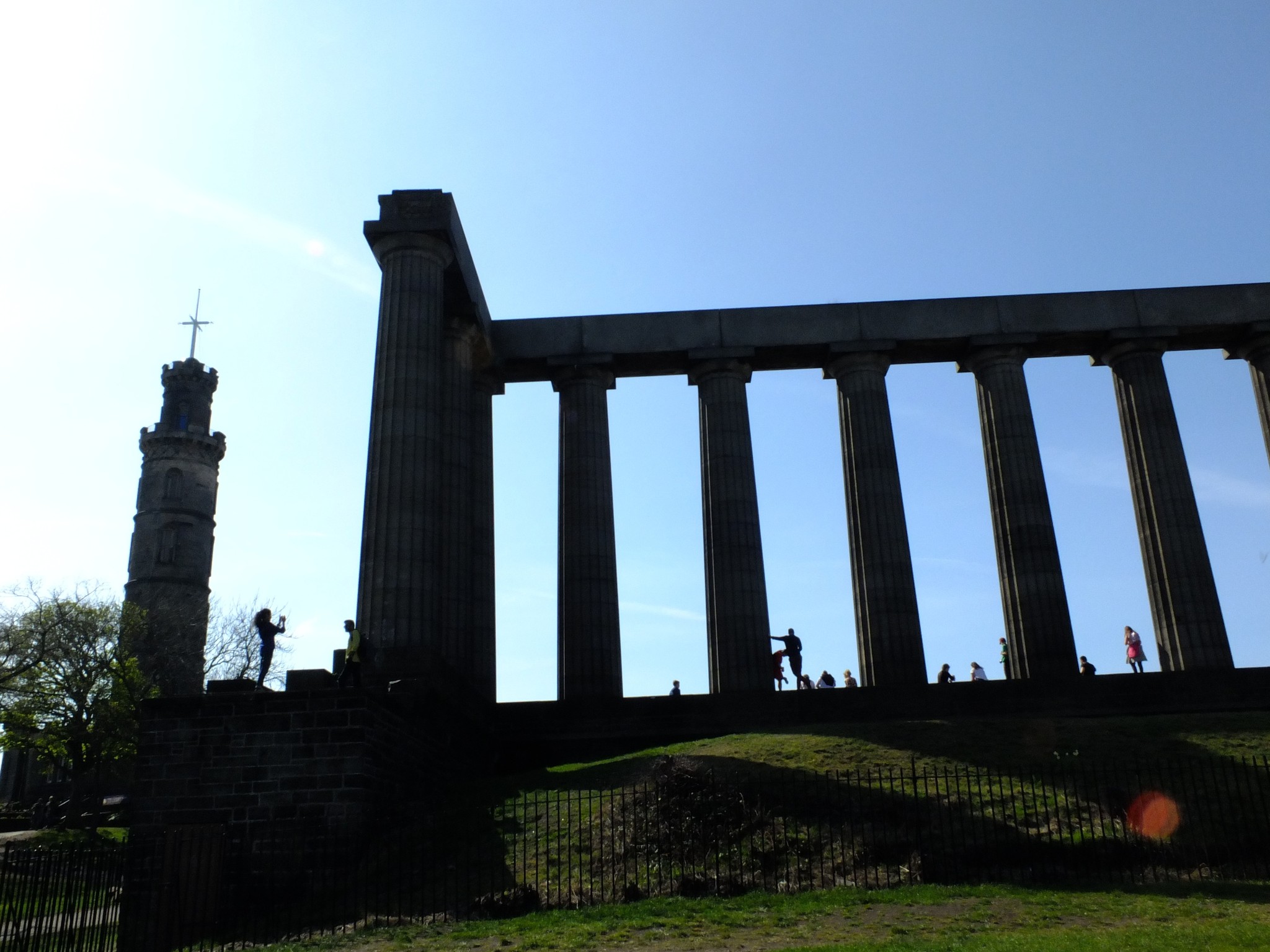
[351,627,373,655]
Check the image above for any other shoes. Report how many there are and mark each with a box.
[784,678,788,684]
[806,680,811,688]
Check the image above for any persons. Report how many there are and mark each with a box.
[253,609,285,692]
[770,629,857,691]
[938,664,955,683]
[338,620,361,689]
[972,663,987,681]
[1126,627,1147,673]
[1080,656,1096,676]
[1000,638,1011,679]
[670,681,681,696]
